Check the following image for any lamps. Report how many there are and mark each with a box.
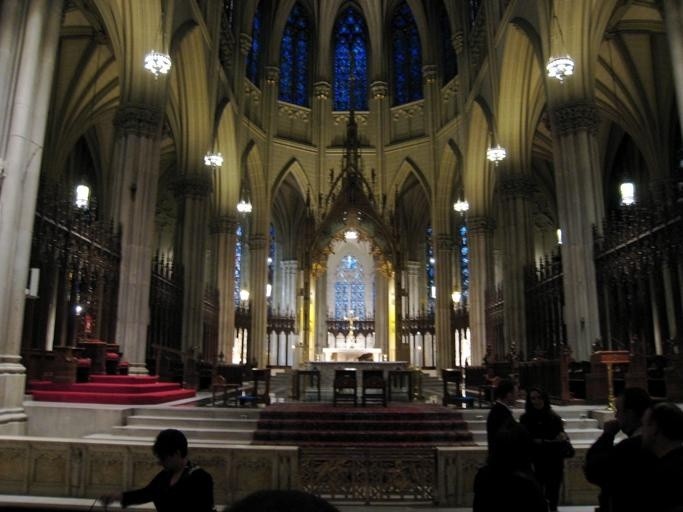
[453,0,507,212]
[143,0,172,79]
[545,1,576,83]
[203,0,253,214]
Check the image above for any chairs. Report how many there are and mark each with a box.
[211,366,501,408]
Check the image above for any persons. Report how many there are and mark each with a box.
[470,375,531,511]
[596,400,682,512]
[96,428,214,511]
[514,385,570,512]
[581,386,656,488]
